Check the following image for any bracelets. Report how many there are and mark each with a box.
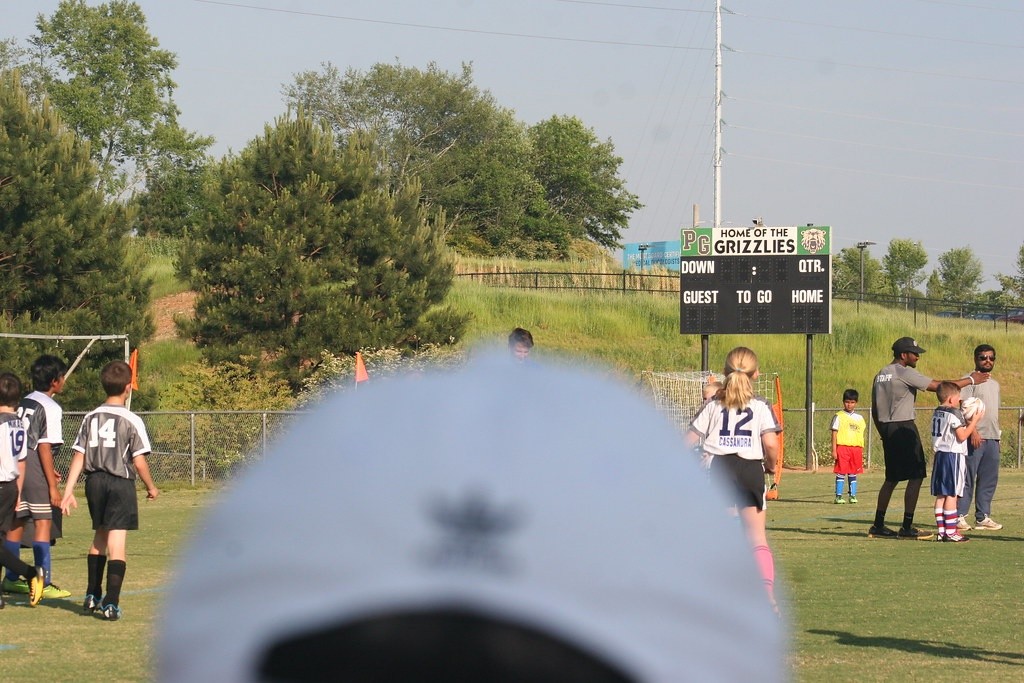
[969,376,975,385]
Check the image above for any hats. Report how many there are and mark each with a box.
[892,337,926,353]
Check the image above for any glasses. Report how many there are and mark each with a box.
[978,354,995,361]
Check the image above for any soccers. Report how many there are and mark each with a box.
[960,397,986,420]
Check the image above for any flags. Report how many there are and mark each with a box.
[130,349,139,390]
[356,353,368,382]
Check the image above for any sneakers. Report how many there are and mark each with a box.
[975,514,1004,530]
[84,594,104,609]
[101,604,121,621]
[41,583,71,599]
[834,495,845,504]
[1,577,31,593]
[868,526,898,539]
[897,528,934,539]
[937,532,969,543]
[849,496,858,503]
[956,514,972,531]
[29,567,43,607]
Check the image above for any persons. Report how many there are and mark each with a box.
[158,345,797,683]
[930,381,984,542]
[61,359,157,621]
[699,381,725,476]
[830,389,866,504]
[684,348,780,610]
[958,344,1004,532]
[867,337,991,539]
[0,355,72,610]
[508,328,534,362]
